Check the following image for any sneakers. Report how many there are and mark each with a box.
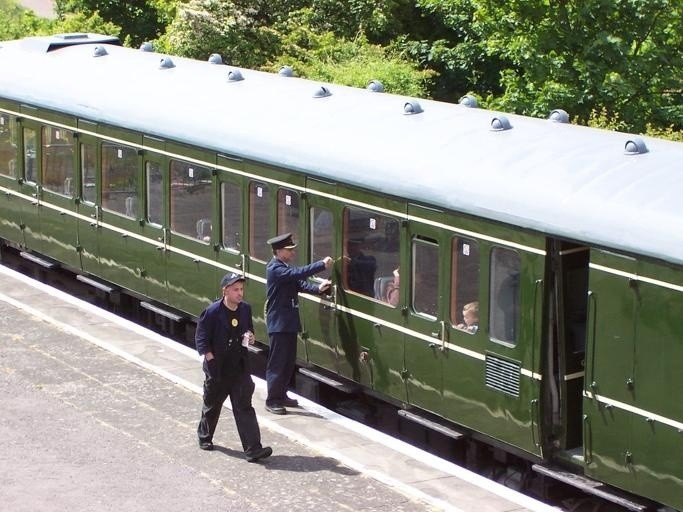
[199,437,213,450]
[246,446,272,462]
[281,398,298,407]
[265,404,287,415]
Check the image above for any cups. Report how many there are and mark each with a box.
[241,332,249,348]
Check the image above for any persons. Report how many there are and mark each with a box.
[265,233,334,414]
[194,272,271,462]
[457,301,480,334]
[385,267,400,306]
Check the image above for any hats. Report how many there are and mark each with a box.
[220,272,246,288]
[267,232,296,250]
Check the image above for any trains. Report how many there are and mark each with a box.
[1,32,683,512]
